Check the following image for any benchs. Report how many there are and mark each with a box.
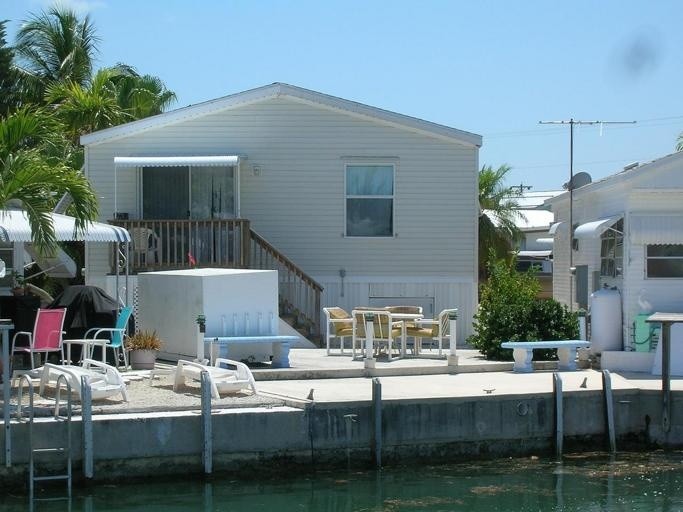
[501,340,591,373]
[203,335,301,369]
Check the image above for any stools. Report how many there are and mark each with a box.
[62,339,110,374]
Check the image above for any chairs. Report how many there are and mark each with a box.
[323,307,364,356]
[80,306,134,370]
[406,308,458,359]
[148,358,258,400]
[12,359,129,402]
[384,306,423,356]
[127,227,162,268]
[9,308,67,377]
[352,307,404,361]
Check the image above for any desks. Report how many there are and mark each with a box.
[391,313,424,358]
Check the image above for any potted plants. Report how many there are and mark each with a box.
[124,329,160,370]
